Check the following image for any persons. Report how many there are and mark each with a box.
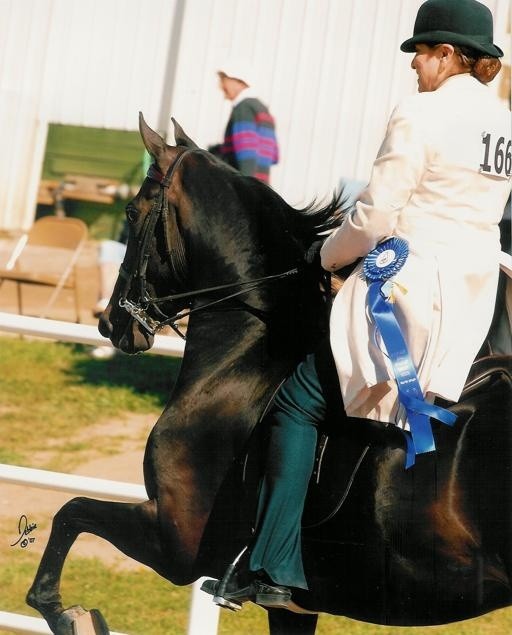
[201,0,512,607]
[211,55,280,187]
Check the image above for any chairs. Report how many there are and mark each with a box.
[2,214,91,323]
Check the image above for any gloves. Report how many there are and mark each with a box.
[303,240,325,264]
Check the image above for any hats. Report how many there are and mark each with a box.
[400,0,504,57]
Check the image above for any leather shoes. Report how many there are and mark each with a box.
[200,571,292,608]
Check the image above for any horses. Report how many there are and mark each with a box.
[25,110,511,635]
